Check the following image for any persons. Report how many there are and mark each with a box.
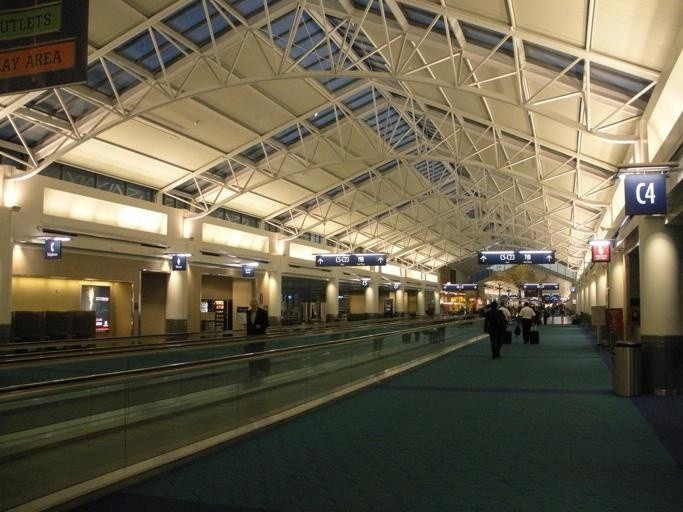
[246,297,270,377]
[482,294,565,359]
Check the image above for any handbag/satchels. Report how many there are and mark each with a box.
[501,331,512,344]
[514,326,521,336]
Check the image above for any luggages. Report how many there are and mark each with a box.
[529,324,539,345]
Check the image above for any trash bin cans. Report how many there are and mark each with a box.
[613,341,643,397]
